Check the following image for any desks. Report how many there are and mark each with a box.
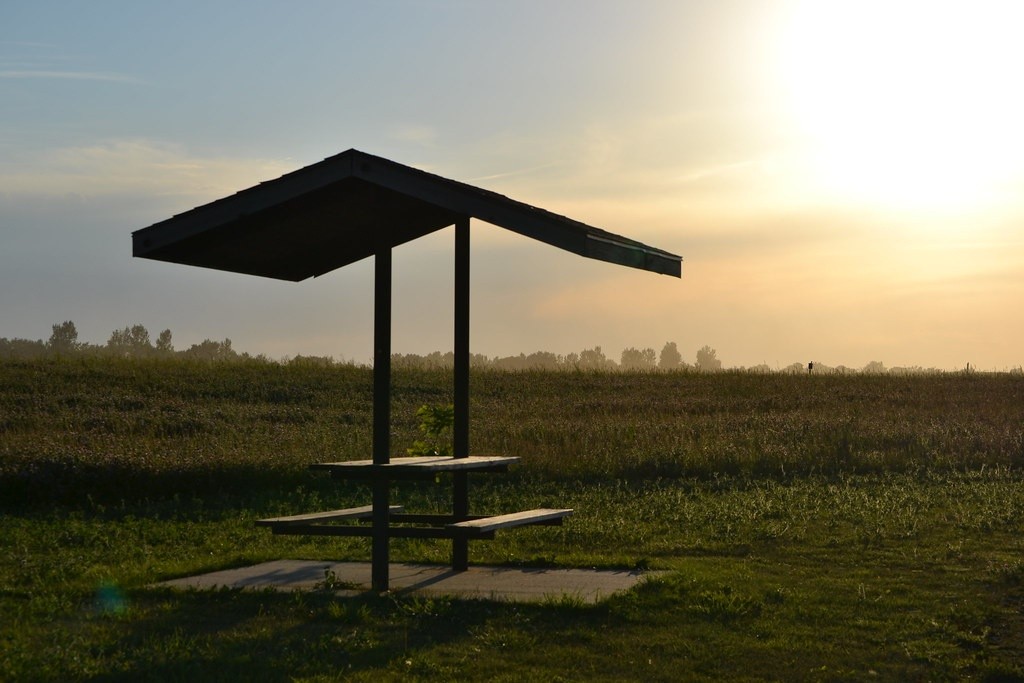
[309,455,519,593]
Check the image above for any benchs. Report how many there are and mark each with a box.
[254,504,401,530]
[447,506,578,533]
